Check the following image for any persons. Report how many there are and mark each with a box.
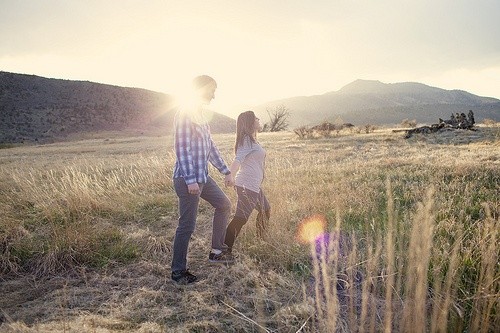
[225,111,271,254]
[171,75,233,285]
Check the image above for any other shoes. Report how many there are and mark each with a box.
[209,250,233,262]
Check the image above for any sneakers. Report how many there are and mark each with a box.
[171,271,196,283]
